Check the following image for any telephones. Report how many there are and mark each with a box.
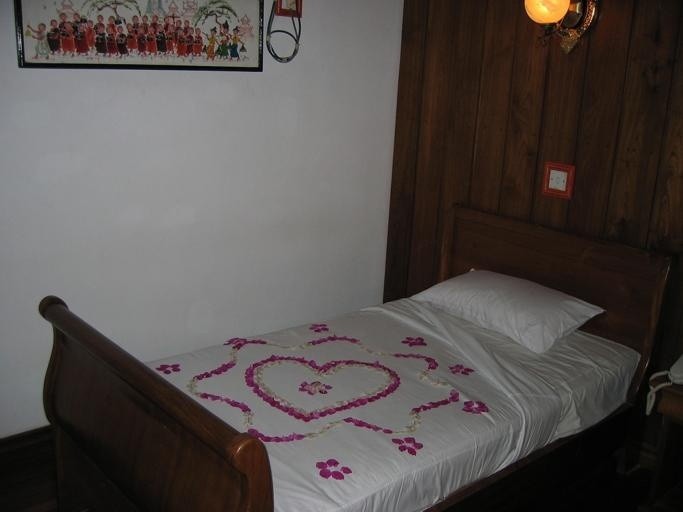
[669,354,683,385]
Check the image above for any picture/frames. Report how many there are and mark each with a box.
[13,0,265,77]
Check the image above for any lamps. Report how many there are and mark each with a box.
[524,0,599,54]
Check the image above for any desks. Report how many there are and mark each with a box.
[646,370,683,505]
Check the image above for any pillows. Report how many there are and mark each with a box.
[406,264,611,356]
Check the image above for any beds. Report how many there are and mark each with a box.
[34,201,673,511]
